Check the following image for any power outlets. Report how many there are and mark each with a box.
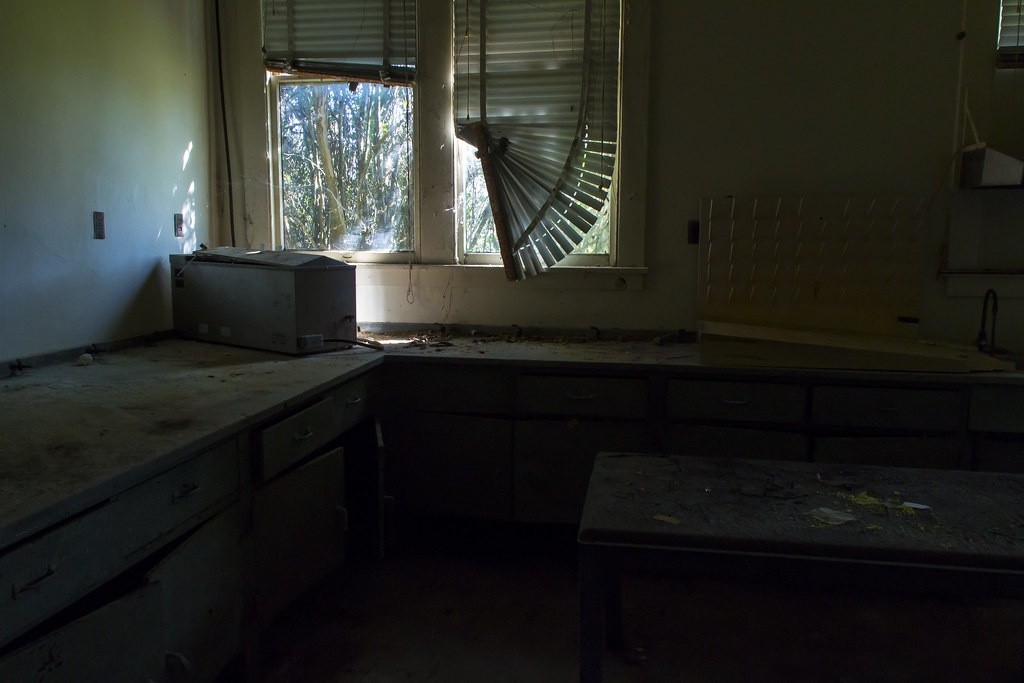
[93,212,106,239]
[174,214,185,236]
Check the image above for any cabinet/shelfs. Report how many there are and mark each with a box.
[0,361,1024,683]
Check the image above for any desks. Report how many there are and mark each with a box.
[574,445,1024,683]
